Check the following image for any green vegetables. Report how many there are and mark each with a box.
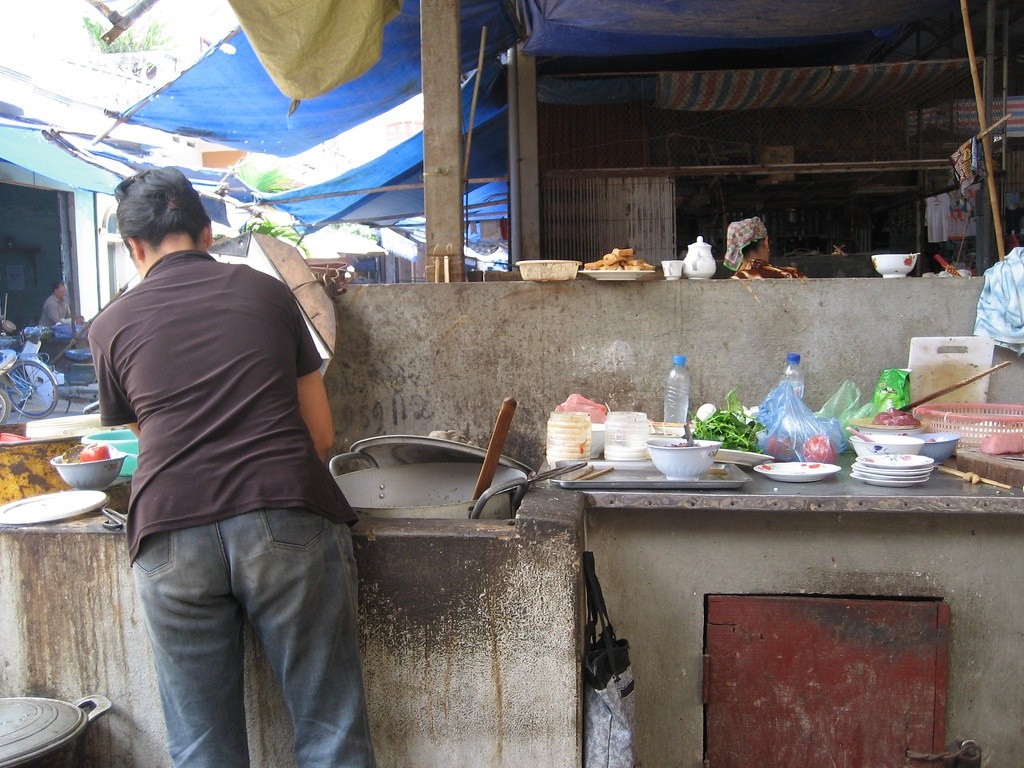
[683,386,765,454]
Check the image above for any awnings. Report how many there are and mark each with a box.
[0,0,501,239]
[524,0,1024,143]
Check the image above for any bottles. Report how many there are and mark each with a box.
[663,356,690,423]
[778,353,805,400]
[604,412,647,461]
[547,412,591,471]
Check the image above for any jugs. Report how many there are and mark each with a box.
[683,235,716,279]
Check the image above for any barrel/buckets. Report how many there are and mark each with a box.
[329,452,528,519]
[0,696,111,768]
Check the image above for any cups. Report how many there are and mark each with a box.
[661,260,684,280]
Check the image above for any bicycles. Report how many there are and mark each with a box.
[5,353,61,423]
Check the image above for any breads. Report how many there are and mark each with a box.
[583,248,657,270]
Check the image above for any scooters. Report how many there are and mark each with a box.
[17,327,100,416]
[1,350,18,424]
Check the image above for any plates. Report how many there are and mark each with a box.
[577,269,655,280]
[849,454,935,487]
[753,462,842,482]
[714,450,775,469]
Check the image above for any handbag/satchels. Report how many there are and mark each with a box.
[755,380,876,463]
[582,549,637,768]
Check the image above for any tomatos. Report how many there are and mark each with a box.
[768,434,800,463]
[802,435,837,464]
[80,442,110,462]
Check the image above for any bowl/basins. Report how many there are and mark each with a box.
[914,434,962,465]
[591,422,606,459]
[81,428,139,476]
[64,348,92,361]
[51,325,85,339]
[50,452,128,492]
[849,435,925,457]
[2,320,17,333]
[857,426,925,437]
[872,254,918,279]
[645,439,722,482]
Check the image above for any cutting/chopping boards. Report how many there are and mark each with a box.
[908,337,994,413]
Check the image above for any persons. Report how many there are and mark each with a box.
[89,169,371,768]
[724,216,809,279]
[38,280,85,326]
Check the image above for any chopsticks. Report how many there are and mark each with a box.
[567,466,613,481]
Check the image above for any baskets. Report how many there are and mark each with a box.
[515,260,582,281]
[913,402,1024,457]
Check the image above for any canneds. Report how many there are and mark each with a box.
[603,410,648,463]
[546,411,591,467]
